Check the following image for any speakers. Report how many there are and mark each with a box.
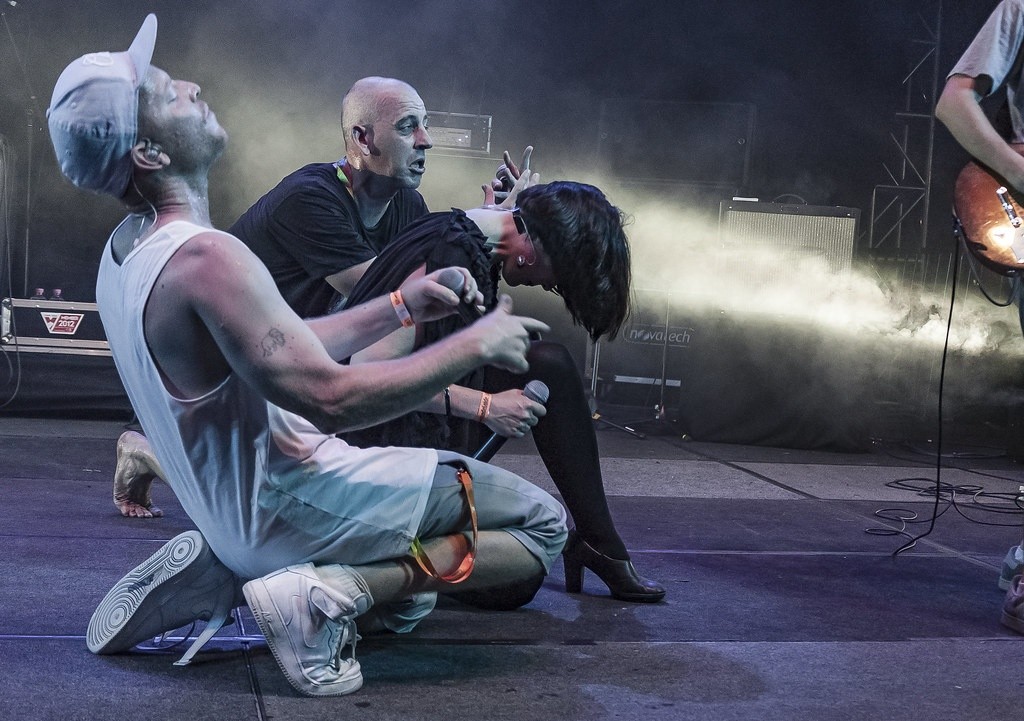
[715,200,862,316]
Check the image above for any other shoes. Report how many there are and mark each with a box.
[999,572,1024,635]
[997,546,1024,590]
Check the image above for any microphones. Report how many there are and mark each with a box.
[473,380,549,463]
[515,216,538,266]
[493,164,510,204]
[437,270,484,327]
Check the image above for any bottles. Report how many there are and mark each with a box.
[49,289,64,301]
[30,288,46,300]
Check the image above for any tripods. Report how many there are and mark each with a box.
[598,296,691,442]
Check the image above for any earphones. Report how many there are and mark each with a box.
[144,142,159,162]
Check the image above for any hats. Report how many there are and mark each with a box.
[46,12,158,199]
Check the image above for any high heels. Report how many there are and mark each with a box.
[562,528,665,601]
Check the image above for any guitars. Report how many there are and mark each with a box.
[954,144,1024,278]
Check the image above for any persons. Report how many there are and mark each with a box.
[113,76,540,518]
[46,12,569,694]
[334,180,666,603]
[935,0,1024,628]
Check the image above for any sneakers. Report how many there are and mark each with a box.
[243,562,371,696]
[85,528,235,666]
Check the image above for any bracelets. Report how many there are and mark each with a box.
[390,289,414,329]
[476,392,493,424]
[445,386,452,418]
